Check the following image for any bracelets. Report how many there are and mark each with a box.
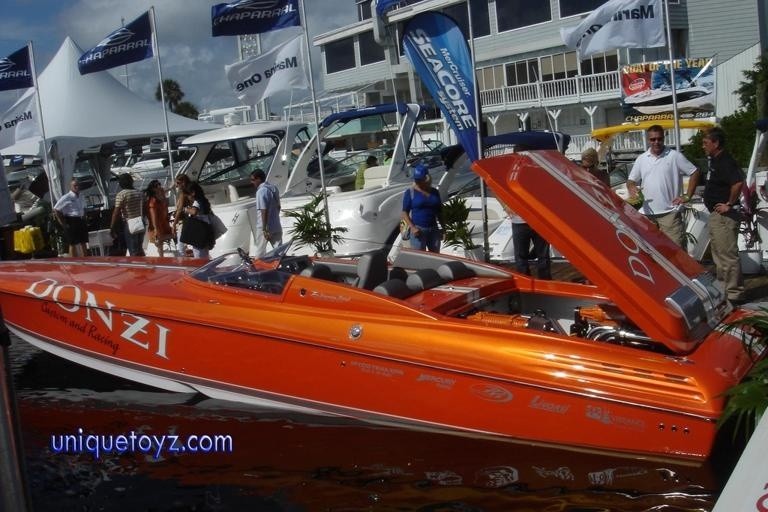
[724,200,732,207]
[684,194,690,200]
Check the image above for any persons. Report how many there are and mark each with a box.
[13,173,215,263]
[353,155,378,191]
[571,147,611,188]
[250,169,283,260]
[382,150,394,165]
[626,125,702,257]
[508,143,551,281]
[701,127,745,308]
[402,165,443,256]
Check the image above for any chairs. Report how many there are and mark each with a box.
[373,278,408,301]
[405,269,440,292]
[352,250,386,291]
[438,262,467,283]
[302,263,329,281]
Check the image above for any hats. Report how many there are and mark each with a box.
[412,165,428,181]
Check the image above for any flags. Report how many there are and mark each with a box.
[77,10,155,76]
[0,44,35,92]
[563,0,667,58]
[211,1,301,38]
[224,36,309,107]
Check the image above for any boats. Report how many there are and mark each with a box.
[14,381,718,512]
[622,68,714,112]
[0,146,767,474]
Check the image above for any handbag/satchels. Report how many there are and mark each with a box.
[387,233,421,264]
[209,214,227,240]
[13,225,45,254]
[128,217,144,234]
[180,217,217,249]
[399,219,411,241]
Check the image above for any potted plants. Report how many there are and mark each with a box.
[439,196,486,265]
[282,189,349,260]
